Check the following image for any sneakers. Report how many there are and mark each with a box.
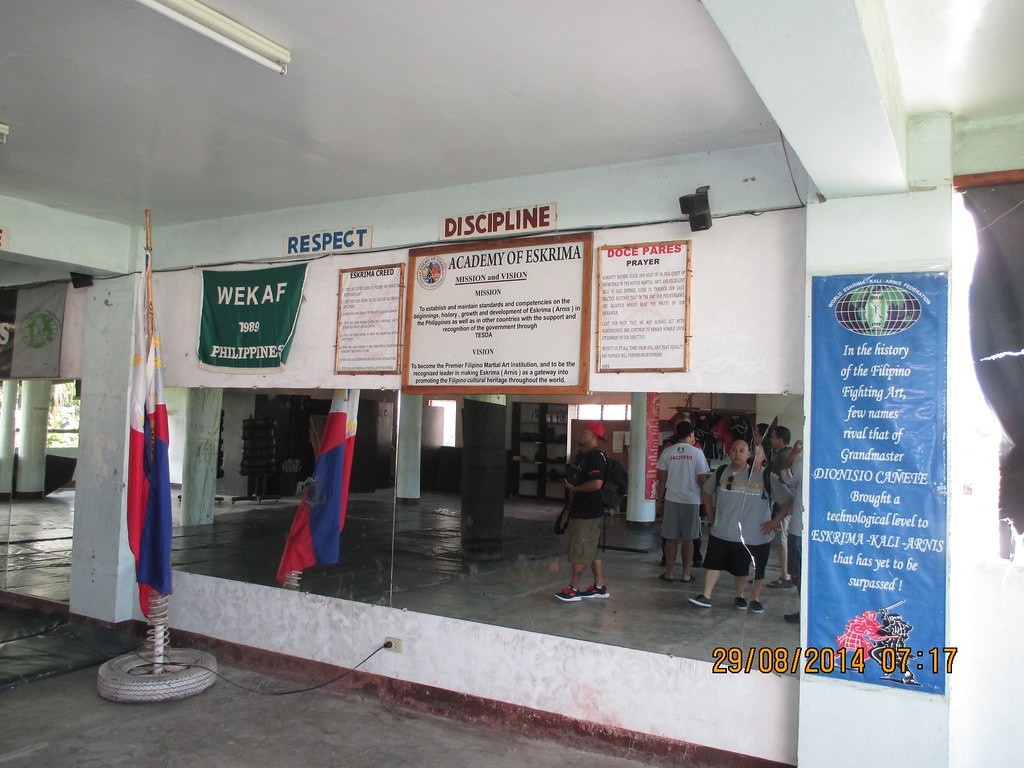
[554,585,581,601]
[688,594,712,608]
[732,596,747,609]
[580,585,610,598]
[751,601,764,613]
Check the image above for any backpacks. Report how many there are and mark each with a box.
[594,452,625,508]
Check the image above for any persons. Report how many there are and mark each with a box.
[554,421,611,603]
[653,413,801,624]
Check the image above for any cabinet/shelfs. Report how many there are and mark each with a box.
[516,422,567,482]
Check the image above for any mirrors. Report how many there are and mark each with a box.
[159,385,803,674]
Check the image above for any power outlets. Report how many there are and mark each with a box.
[384,637,403,651]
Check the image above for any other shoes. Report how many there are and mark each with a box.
[694,561,702,568]
[784,613,800,623]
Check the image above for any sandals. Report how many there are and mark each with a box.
[767,577,793,588]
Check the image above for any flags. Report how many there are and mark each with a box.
[277,390,361,585]
[125,255,173,617]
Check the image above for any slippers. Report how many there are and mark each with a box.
[660,573,673,581]
[682,575,694,583]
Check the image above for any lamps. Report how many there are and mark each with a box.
[133,0,290,77]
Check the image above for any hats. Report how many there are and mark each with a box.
[676,421,693,438]
[585,421,607,441]
